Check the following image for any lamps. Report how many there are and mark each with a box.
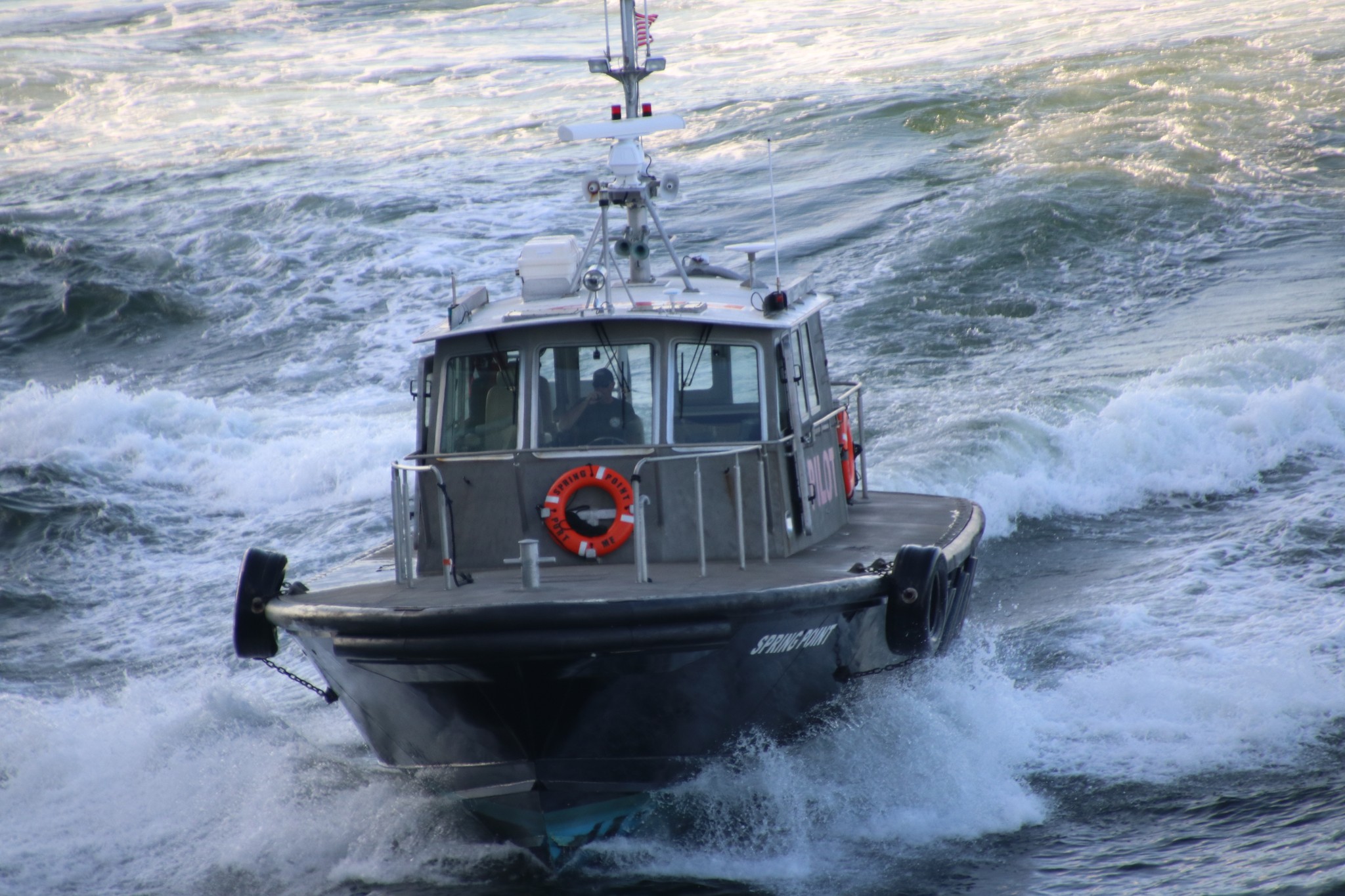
[583,264,607,306]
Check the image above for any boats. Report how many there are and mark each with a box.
[234,1,985,787]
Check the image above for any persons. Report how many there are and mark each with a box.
[555,369,651,448]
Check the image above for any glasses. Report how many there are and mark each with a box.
[592,379,612,387]
